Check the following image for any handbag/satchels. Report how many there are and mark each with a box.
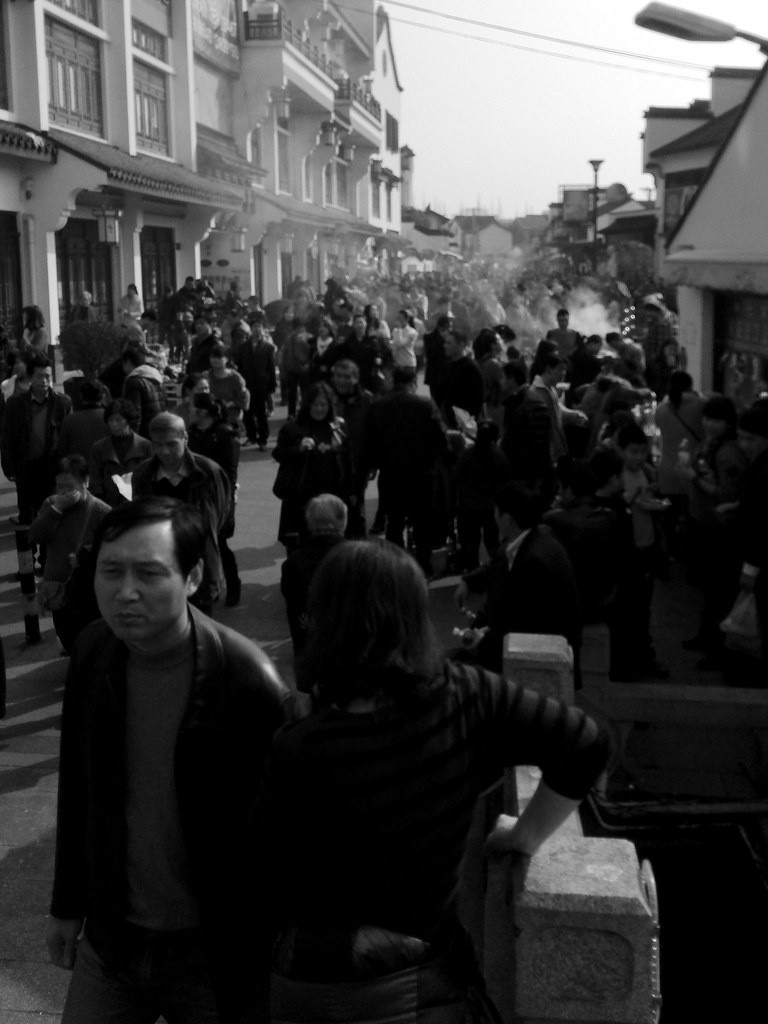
[274,461,307,499]
[37,581,66,612]
[720,590,758,639]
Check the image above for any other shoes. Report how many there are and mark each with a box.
[261,445,266,451]
[225,580,241,606]
[243,440,254,446]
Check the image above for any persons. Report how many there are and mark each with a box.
[0,269,768,1024]
[46,502,308,1024]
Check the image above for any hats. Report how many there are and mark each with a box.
[739,398,768,439]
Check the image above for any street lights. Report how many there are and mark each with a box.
[590,159,604,276]
